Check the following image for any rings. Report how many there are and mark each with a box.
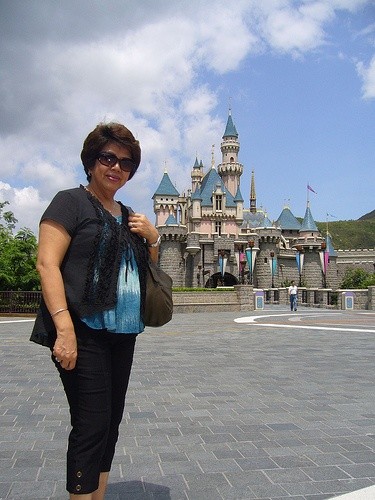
[56,357,61,362]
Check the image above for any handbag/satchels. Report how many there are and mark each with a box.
[124,206,173,328]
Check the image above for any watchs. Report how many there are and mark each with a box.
[146,234,162,247]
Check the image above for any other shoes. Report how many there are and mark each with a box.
[295,309,297,311]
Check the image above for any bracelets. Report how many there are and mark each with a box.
[51,308,68,317]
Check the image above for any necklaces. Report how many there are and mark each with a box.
[109,201,117,213]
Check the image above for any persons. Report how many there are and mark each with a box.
[288,279,298,311]
[28,113,161,500]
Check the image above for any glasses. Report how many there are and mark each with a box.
[97,152,136,172]
